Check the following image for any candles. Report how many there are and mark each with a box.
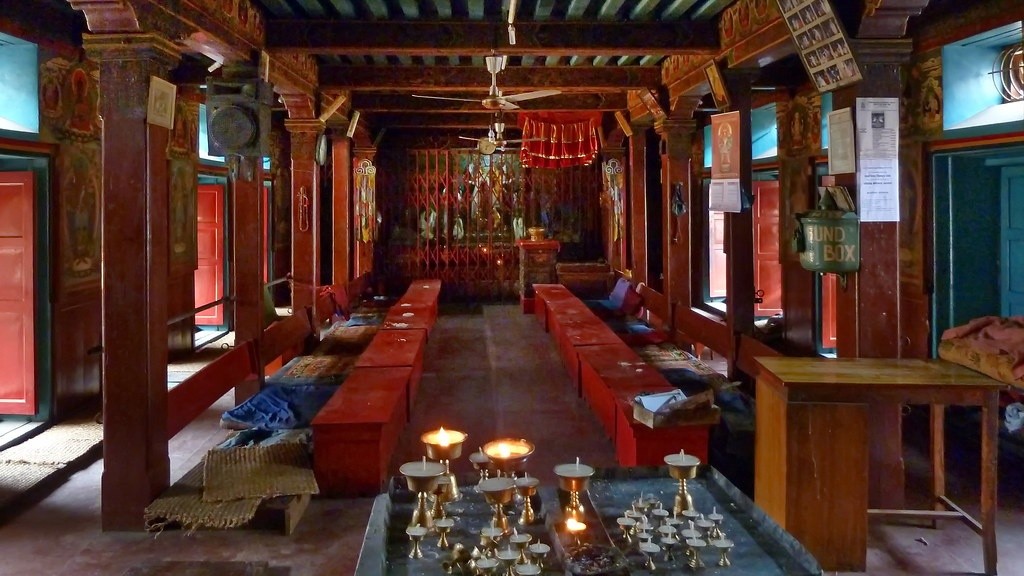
[400,426,735,576]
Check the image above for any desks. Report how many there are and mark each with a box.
[747,357,1010,575]
[534,284,708,466]
[311,280,441,496]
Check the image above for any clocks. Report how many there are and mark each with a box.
[477,136,497,155]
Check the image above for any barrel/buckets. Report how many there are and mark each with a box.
[528,226,545,241]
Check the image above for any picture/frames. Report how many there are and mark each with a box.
[614,111,634,137]
[145,76,177,129]
[638,87,668,123]
[347,110,360,137]
[702,58,732,111]
[777,0,863,95]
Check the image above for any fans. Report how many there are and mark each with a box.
[411,55,563,152]
